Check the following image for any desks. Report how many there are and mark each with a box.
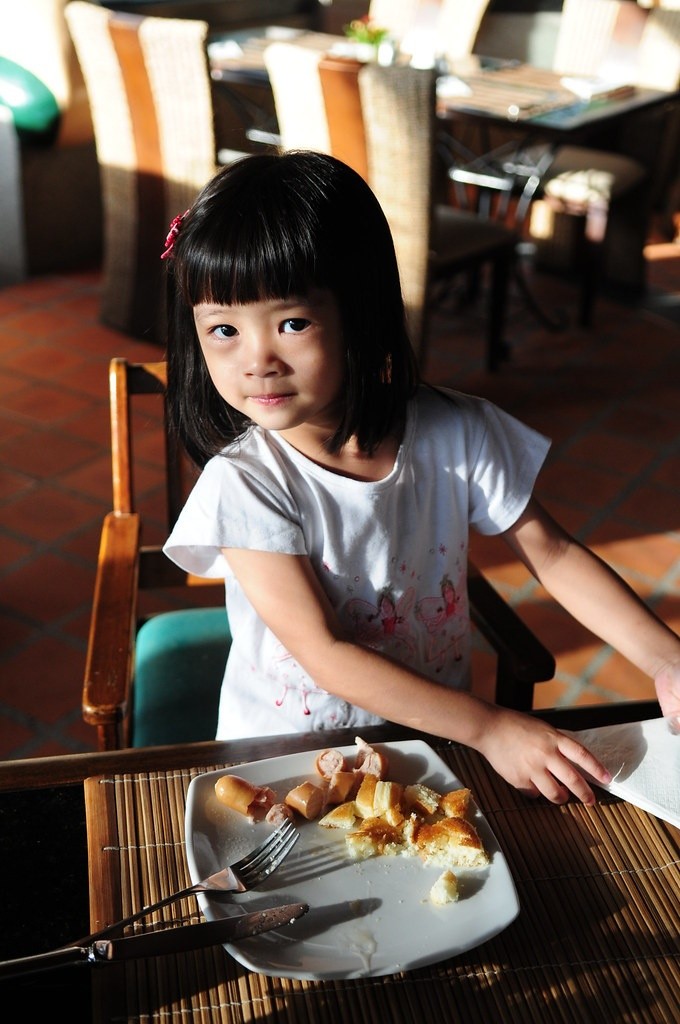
[204,24,680,375]
[0,699,680,1024]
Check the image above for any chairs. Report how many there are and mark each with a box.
[78,355,558,750]
[66,1,680,375]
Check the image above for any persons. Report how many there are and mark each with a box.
[163,149,680,806]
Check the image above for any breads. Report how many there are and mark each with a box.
[317,773,488,908]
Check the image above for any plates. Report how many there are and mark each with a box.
[185,739,522,982]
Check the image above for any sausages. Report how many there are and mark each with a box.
[214,736,388,829]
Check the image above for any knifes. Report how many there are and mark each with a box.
[0,902,310,981]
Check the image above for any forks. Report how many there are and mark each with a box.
[68,816,300,948]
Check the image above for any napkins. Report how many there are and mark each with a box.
[559,718,680,830]
